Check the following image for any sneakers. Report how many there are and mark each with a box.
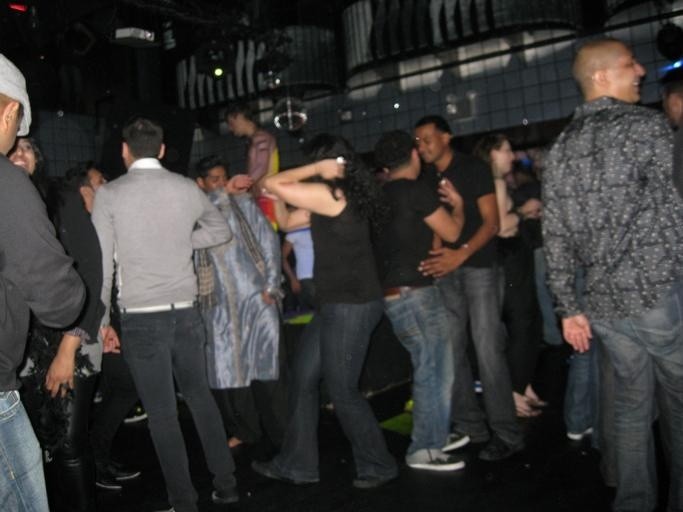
[408,451,467,473]
[251,460,323,483]
[354,470,396,488]
[122,408,151,425]
[207,489,240,504]
[42,447,80,468]
[96,466,141,490]
[479,440,527,462]
[440,431,470,451]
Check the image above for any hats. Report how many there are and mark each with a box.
[0,51,33,135]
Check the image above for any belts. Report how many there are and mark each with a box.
[382,282,435,300]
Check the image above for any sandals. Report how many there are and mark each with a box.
[511,397,549,419]
